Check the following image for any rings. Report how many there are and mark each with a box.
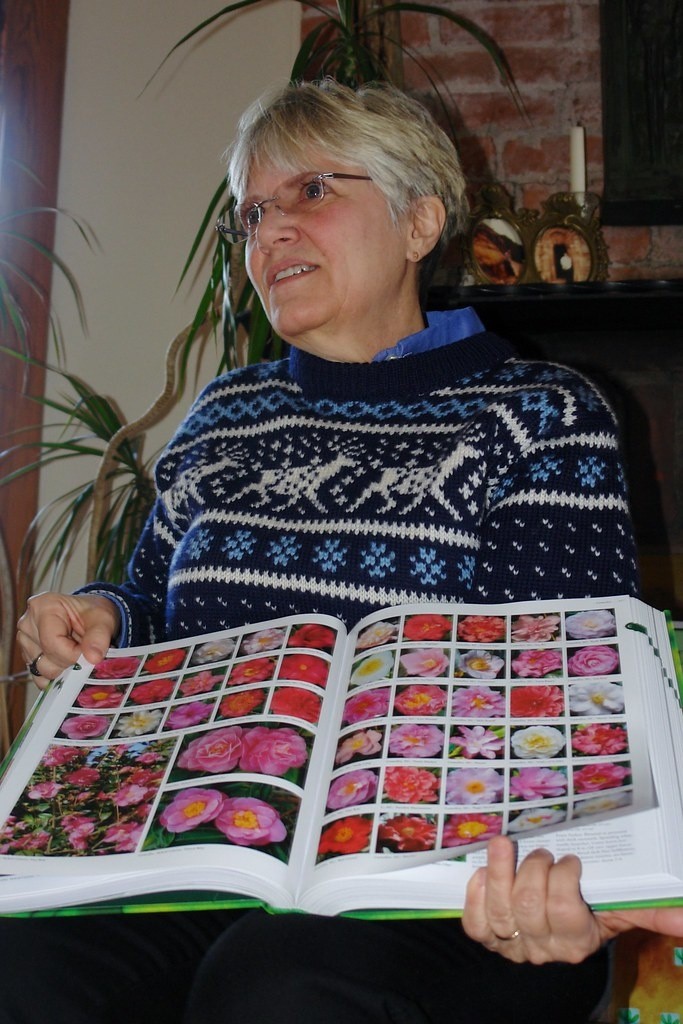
[496,930,519,941]
[30,652,44,676]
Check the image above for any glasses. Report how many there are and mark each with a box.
[213,171,373,244]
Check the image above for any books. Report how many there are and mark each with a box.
[0,594,683,920]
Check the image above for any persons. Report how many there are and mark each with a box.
[0,76,683,1024]
